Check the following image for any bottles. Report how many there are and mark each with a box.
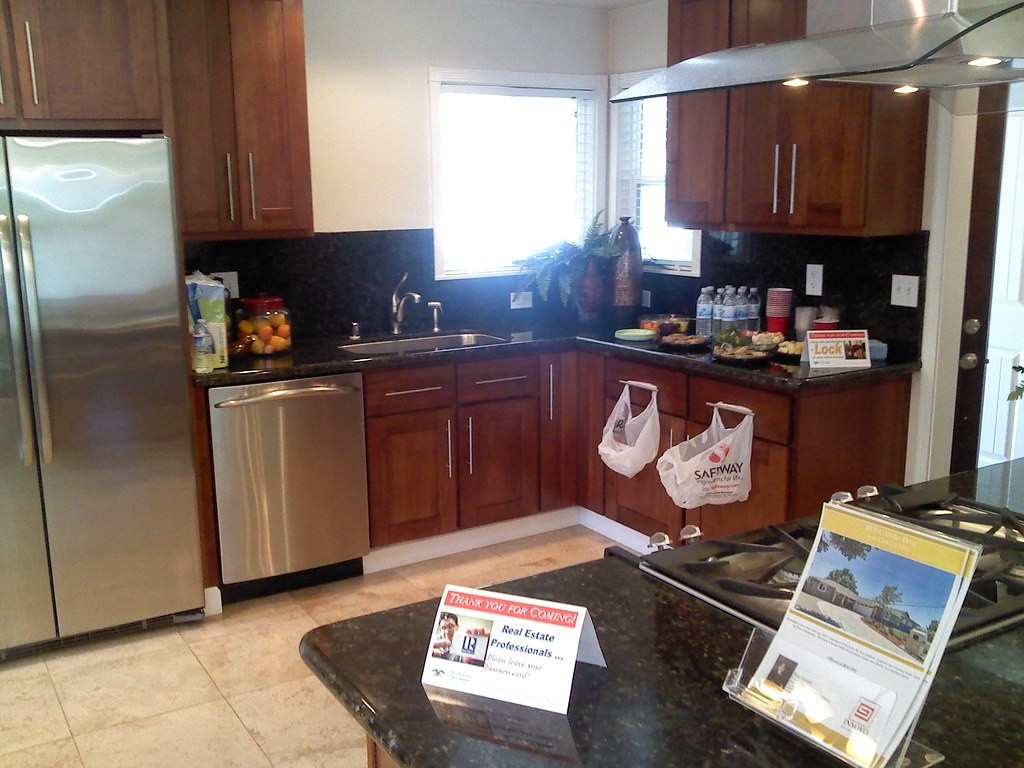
[722,285,736,330]
[193,319,214,373]
[712,288,725,338]
[696,286,715,338]
[748,288,760,333]
[659,314,664,323]
[740,285,749,295]
[213,277,233,354]
[736,289,748,330]
[670,314,675,323]
[236,292,291,354]
[609,217,642,327]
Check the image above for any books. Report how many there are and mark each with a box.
[740,497,983,768]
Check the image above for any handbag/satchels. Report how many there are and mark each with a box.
[597,379,662,479]
[655,402,755,509]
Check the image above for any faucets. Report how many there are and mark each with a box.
[386,271,424,335]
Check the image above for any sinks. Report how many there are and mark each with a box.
[337,332,510,355]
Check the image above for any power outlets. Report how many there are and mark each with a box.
[641,289,651,308]
[211,271,240,299]
[805,264,823,297]
[510,292,533,310]
[890,274,920,308]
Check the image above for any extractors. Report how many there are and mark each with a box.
[609,0,1024,116]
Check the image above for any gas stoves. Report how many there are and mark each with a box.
[639,484,1024,655]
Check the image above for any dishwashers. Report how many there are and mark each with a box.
[208,372,370,606]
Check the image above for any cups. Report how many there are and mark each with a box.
[814,319,840,330]
[680,322,689,332]
[765,287,793,341]
[795,307,818,342]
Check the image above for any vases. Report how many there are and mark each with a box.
[611,214,644,307]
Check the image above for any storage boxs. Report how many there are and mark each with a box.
[184,274,229,372]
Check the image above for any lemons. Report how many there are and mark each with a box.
[238,311,291,354]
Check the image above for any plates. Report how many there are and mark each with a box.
[774,350,802,358]
[615,329,657,341]
[659,339,707,351]
[713,351,774,364]
[713,341,778,351]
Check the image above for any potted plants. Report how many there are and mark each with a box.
[508,209,621,321]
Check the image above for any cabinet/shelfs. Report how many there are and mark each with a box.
[358,348,914,559]
[665,0,933,235]
[0,0,314,243]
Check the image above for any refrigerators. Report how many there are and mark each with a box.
[1,136,206,650]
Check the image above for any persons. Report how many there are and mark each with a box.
[770,662,786,689]
[433,612,491,663]
